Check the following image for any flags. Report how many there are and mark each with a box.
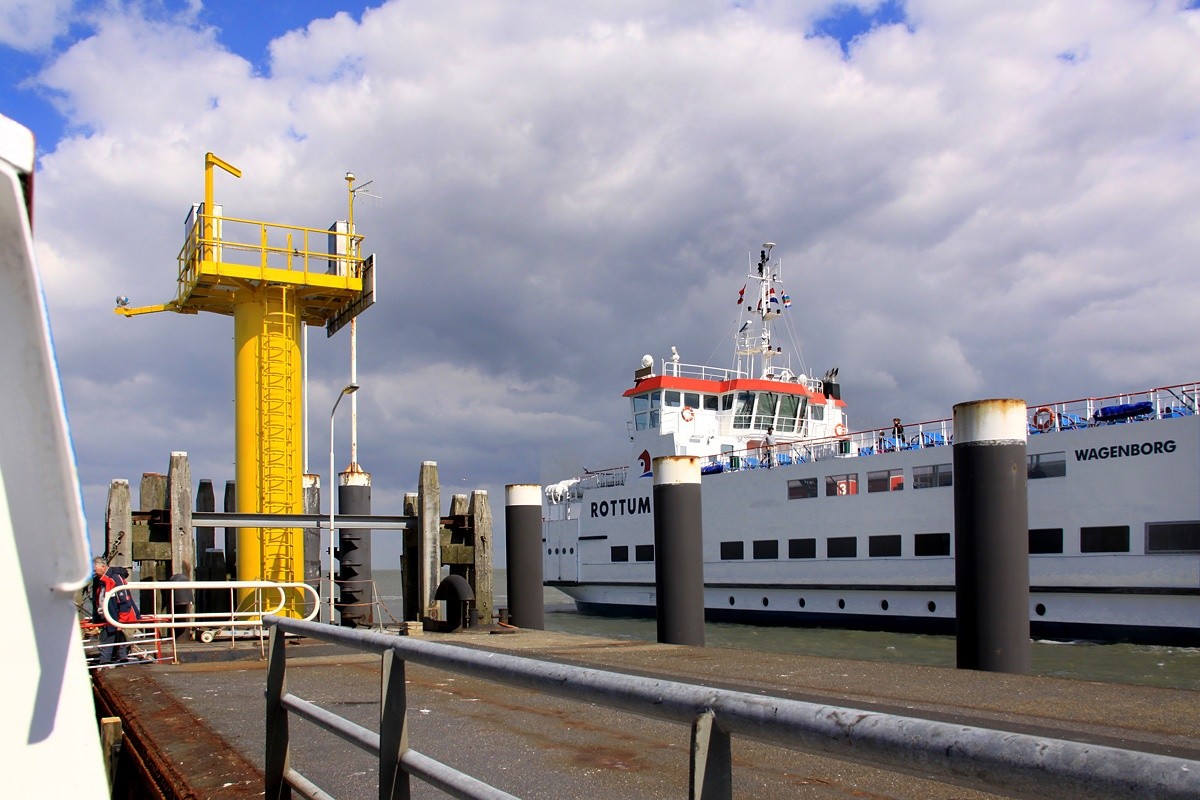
[736,285,745,303]
[781,292,793,308]
[757,287,779,308]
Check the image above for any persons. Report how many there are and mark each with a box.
[892,418,906,443]
[91,556,142,668]
[760,428,776,469]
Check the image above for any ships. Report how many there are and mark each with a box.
[539,243,1200,649]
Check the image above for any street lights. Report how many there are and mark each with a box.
[329,382,361,625]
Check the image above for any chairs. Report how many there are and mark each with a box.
[858,432,953,456]
[1029,401,1193,435]
[701,454,806,475]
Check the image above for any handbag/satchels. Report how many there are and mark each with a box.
[902,434,905,442]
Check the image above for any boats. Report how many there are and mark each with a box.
[1092,400,1153,421]
